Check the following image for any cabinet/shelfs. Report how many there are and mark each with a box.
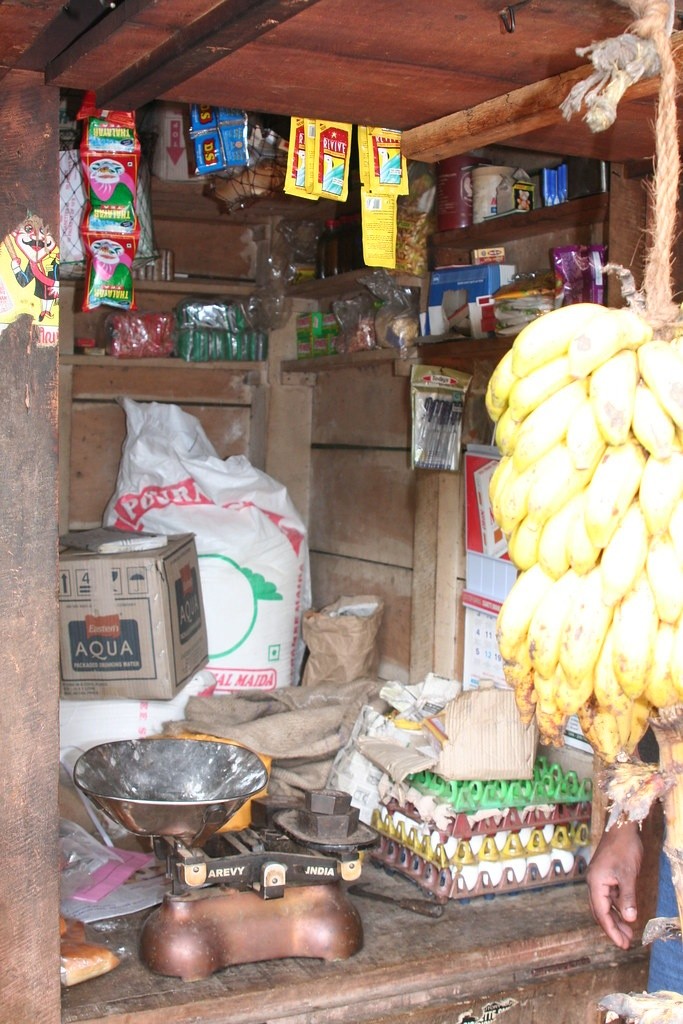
[45,191,610,392]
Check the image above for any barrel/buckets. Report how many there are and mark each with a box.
[436,154,493,228]
[469,164,516,223]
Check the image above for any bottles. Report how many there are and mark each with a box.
[315,213,363,281]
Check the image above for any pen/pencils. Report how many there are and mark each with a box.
[414,397,464,472]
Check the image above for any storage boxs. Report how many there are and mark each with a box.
[496,168,537,215]
[56,531,208,701]
[150,107,220,182]
[420,264,518,335]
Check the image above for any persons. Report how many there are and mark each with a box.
[585,746,683,994]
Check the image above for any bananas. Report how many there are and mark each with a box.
[485,302,683,761]
